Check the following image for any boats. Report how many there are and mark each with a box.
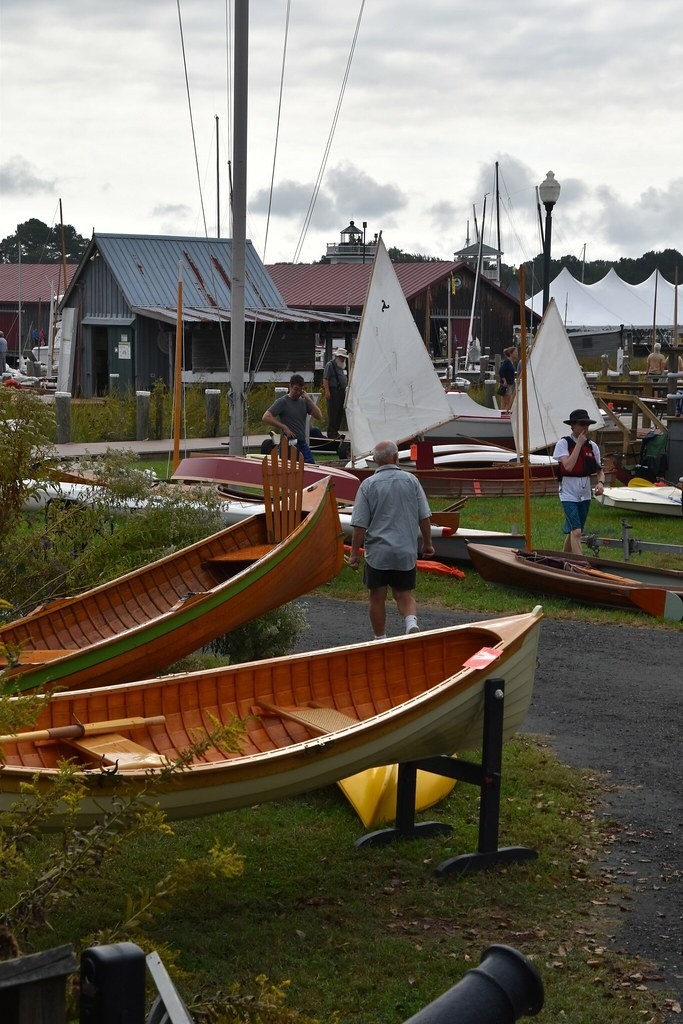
[0,479,346,702]
[464,541,683,625]
[339,753,464,829]
[0,605,545,829]
[169,452,361,510]
[11,477,532,565]
[591,476,683,520]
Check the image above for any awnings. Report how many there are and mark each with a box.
[133,306,362,344]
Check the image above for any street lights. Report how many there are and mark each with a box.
[362,221,368,263]
[537,169,562,319]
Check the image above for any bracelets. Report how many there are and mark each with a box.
[599,480,605,486]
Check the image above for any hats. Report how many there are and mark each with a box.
[332,348,348,358]
[563,409,597,424]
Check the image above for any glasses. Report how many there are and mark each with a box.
[574,423,590,426]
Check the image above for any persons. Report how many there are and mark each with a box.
[553,409,605,554]
[500,345,532,410]
[322,348,349,438]
[348,441,435,640]
[262,375,323,463]
[0,331,8,375]
[646,343,683,375]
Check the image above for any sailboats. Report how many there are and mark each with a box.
[1,240,58,388]
[332,230,605,477]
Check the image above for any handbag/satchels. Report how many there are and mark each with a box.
[497,385,506,396]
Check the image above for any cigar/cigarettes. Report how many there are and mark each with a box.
[585,431,592,434]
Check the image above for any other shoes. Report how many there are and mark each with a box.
[329,433,345,440]
[406,623,420,634]
